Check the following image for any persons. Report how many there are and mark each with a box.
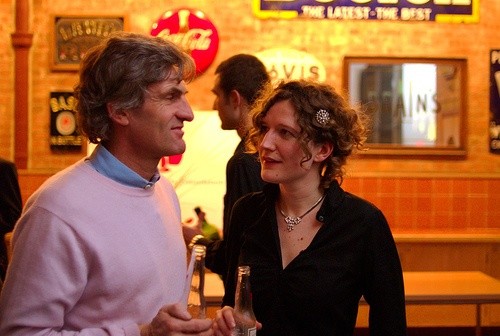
[0,34,218,336]
[0,158,23,285]
[212,80,407,336]
[182,53,271,278]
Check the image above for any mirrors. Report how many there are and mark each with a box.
[340,54,473,161]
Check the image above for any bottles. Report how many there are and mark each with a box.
[194,207,221,244]
[175,245,209,321]
[225,266,258,336]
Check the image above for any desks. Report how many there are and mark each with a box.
[203,268,500,336]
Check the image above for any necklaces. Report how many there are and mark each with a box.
[279,190,324,231]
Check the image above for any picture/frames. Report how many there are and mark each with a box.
[48,11,131,74]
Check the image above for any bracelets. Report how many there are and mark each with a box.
[189,235,204,250]
